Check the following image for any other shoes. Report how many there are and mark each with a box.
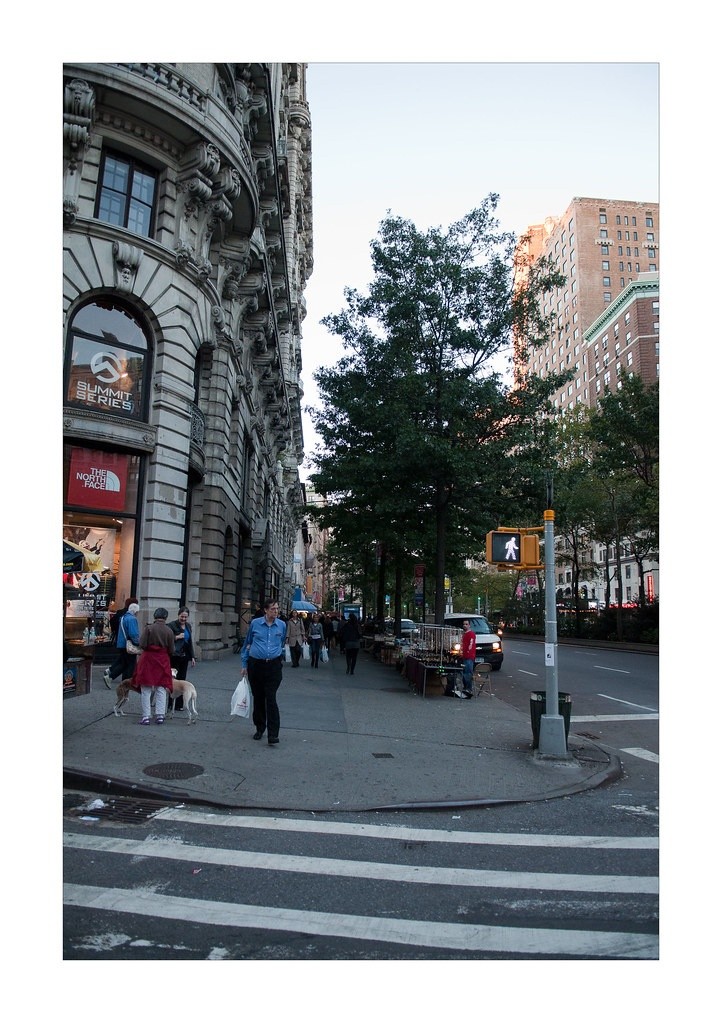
[103,675,112,689]
[296,663,299,667]
[167,708,171,712]
[154,715,164,724]
[346,667,350,673]
[253,731,263,740]
[311,659,315,667]
[104,669,111,675]
[172,705,183,711]
[461,687,473,694]
[315,662,318,668]
[268,736,279,744]
[137,716,151,725]
[350,668,354,674]
[293,664,296,668]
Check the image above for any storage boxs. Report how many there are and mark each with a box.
[426,679,444,696]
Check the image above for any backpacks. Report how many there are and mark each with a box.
[110,608,127,632]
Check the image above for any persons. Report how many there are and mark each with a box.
[277,610,362,675]
[461,620,476,693]
[240,598,286,746]
[103,598,140,689]
[165,606,196,711]
[92,598,115,638]
[131,607,177,725]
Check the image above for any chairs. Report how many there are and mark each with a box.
[473,662,492,699]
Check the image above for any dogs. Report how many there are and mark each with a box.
[114,678,132,716]
[166,668,199,725]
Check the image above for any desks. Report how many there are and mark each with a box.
[419,662,463,697]
[406,655,438,686]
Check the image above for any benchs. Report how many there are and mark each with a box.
[235,627,246,653]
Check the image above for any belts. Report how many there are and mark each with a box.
[249,657,282,664]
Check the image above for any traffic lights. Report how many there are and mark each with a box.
[523,534,540,565]
[486,530,523,565]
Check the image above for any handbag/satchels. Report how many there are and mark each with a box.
[230,674,251,719]
[285,644,292,662]
[320,642,329,664]
[126,640,142,655]
[169,621,185,657]
[307,624,313,645]
[303,642,310,660]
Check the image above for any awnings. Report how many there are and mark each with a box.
[291,601,317,611]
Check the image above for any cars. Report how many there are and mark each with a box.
[385,617,420,638]
[488,622,502,641]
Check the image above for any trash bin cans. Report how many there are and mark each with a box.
[529,690,572,749]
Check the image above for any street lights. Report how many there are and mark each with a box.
[582,587,599,618]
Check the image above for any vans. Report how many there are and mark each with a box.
[425,613,504,671]
